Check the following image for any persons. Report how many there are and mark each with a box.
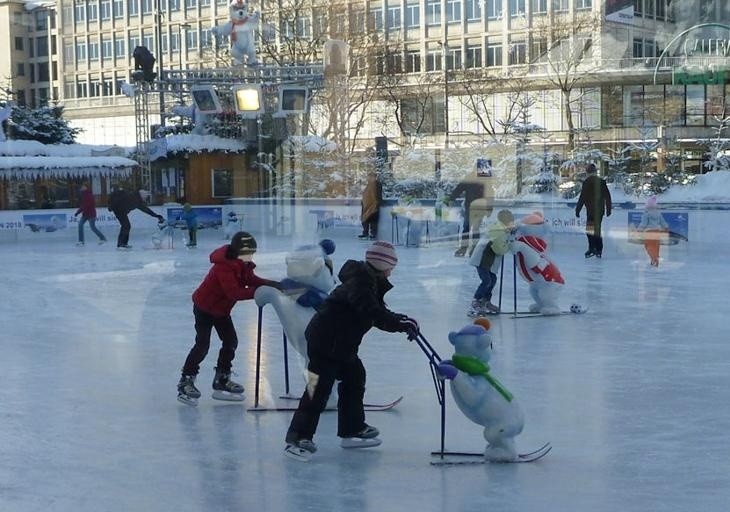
[108,189,164,249]
[175,202,197,246]
[133,46,157,90]
[75,182,107,245]
[637,195,669,265]
[177,232,288,399]
[286,241,421,453]
[448,163,495,256]
[468,209,514,312]
[575,164,612,255]
[358,173,382,239]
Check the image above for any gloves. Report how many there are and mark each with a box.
[399,315,418,342]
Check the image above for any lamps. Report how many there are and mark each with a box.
[279,86,308,114]
[190,84,224,114]
[232,84,265,115]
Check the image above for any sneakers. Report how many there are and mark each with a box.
[212,367,244,394]
[176,372,202,399]
[454,246,467,254]
[358,234,376,239]
[117,243,132,249]
[186,242,197,246]
[585,249,602,256]
[286,438,317,454]
[468,299,499,315]
[339,423,379,440]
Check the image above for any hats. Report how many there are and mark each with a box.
[645,198,657,206]
[498,209,515,225]
[183,203,192,211]
[366,240,399,270]
[586,164,596,172]
[230,231,257,258]
